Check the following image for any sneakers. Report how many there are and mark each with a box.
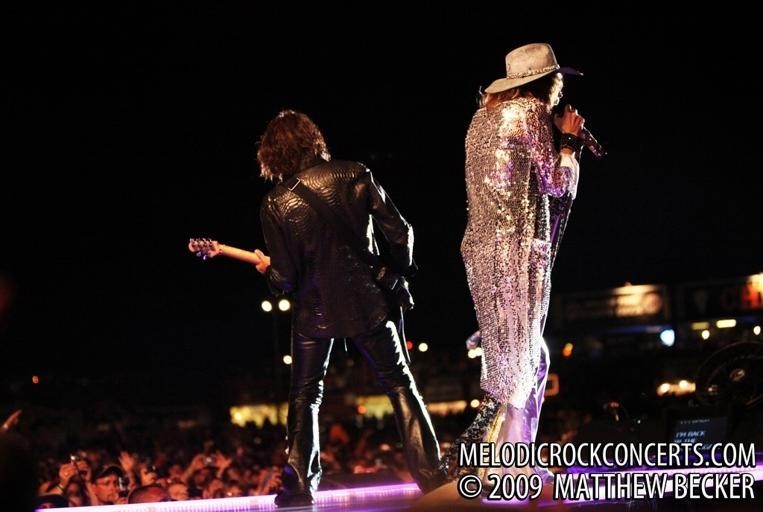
[274,495,314,508]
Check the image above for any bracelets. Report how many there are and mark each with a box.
[559,133,582,152]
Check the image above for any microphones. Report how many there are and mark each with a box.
[555,102,608,161]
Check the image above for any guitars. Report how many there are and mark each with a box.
[188,238,415,309]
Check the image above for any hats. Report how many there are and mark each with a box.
[484,42,584,94]
[38,495,68,507]
[93,464,123,477]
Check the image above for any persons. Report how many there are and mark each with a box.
[420,42,586,494]
[255,110,443,506]
[0,411,452,510]
